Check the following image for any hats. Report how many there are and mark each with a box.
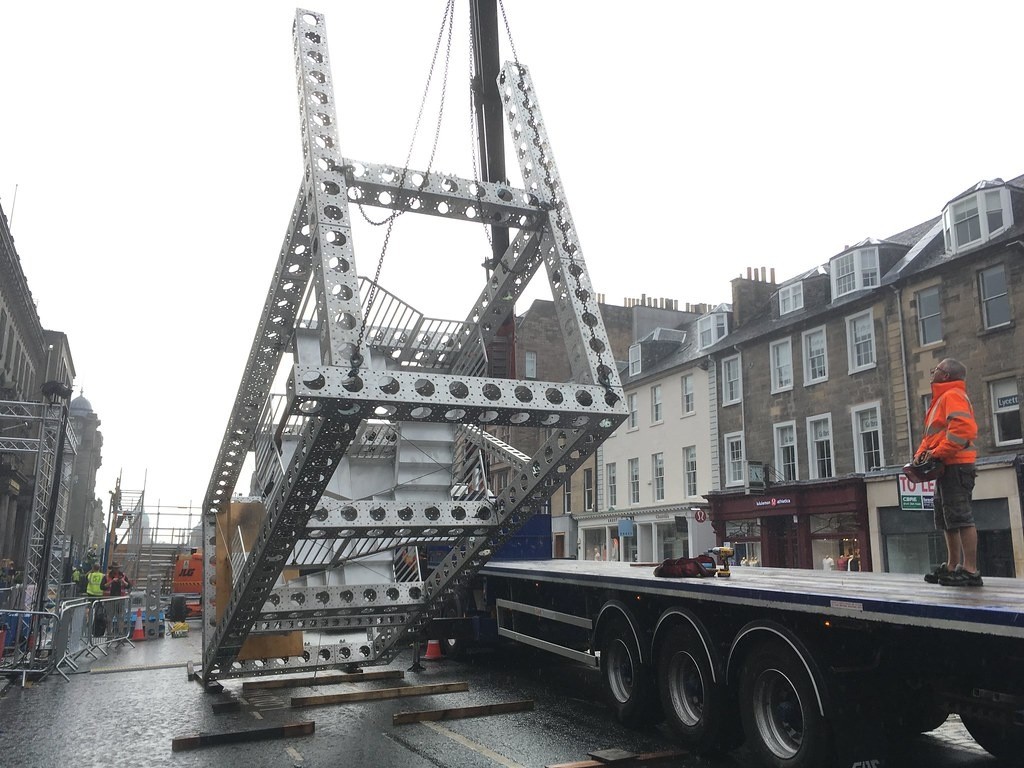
[108,561,123,568]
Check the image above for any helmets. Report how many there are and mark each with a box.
[903,456,945,481]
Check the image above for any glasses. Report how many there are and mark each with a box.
[933,366,950,377]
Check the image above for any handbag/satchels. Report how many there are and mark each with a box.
[92,603,108,636]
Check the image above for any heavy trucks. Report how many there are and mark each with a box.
[400,490,1024,768]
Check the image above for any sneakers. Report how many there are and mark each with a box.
[924,561,963,583]
[938,563,984,588]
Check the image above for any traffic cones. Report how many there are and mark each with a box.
[131,608,148,641]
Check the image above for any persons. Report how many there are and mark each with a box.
[740,556,759,567]
[85,564,104,626]
[731,559,737,565]
[595,548,600,560]
[72,544,98,588]
[837,550,861,571]
[100,561,130,637]
[913,359,984,585]
[0,559,15,606]
[823,555,834,571]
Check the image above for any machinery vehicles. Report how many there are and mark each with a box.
[170,548,204,622]
